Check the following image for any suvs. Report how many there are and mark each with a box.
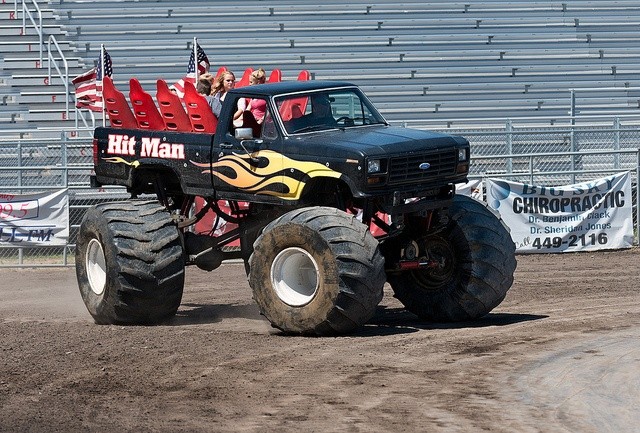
[76,80,516,333]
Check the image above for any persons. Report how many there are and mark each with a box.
[198,73,214,85]
[195,79,222,119]
[209,71,247,129]
[233,70,272,127]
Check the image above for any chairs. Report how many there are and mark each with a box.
[242,110,263,137]
[214,67,227,79]
[232,68,254,106]
[183,80,218,134]
[278,70,309,122]
[128,79,167,131]
[103,77,138,129]
[265,69,280,123]
[291,104,304,120]
[156,79,193,132]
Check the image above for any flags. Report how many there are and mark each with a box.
[169,43,210,115]
[72,48,113,113]
